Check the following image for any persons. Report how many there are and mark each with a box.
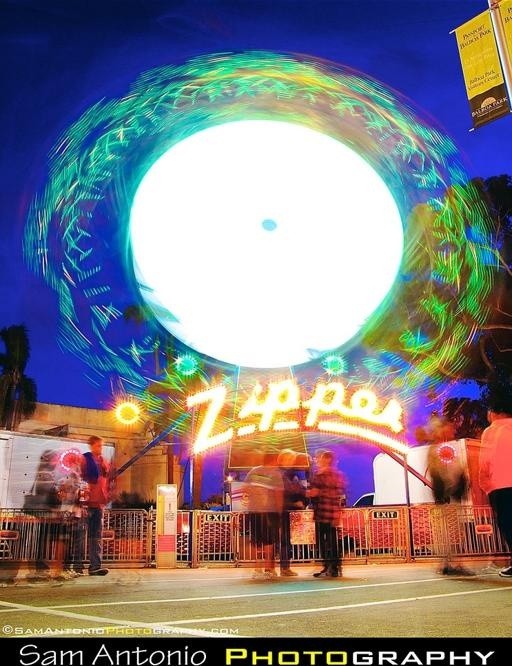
[33,449,75,572]
[72,434,110,576]
[236,446,348,579]
[477,401,512,580]
[426,418,475,577]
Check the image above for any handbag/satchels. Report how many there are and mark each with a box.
[22,470,55,517]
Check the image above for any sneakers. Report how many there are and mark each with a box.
[498,566,511,576]
[252,569,298,581]
[313,567,343,578]
[88,568,109,576]
